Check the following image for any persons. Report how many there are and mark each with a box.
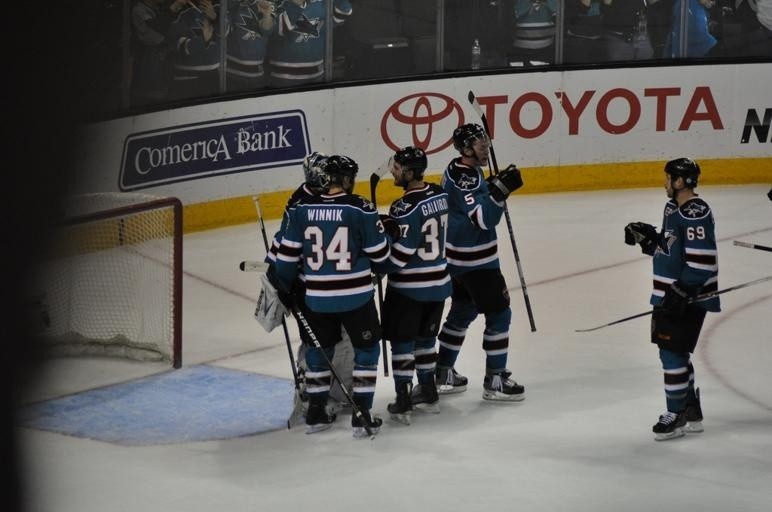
[623,156,721,441]
[170,1,230,78]
[435,124,527,401]
[267,1,353,90]
[263,152,355,420]
[129,0,188,48]
[505,0,772,61]
[219,0,277,83]
[374,146,454,425]
[276,155,392,441]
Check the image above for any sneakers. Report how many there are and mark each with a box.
[659,401,703,421]
[484,371,525,394]
[305,404,336,425]
[653,413,687,433]
[351,410,382,428]
[411,385,439,403]
[387,395,413,413]
[436,367,468,387]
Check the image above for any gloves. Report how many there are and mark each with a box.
[486,175,496,184]
[624,222,659,252]
[490,164,523,201]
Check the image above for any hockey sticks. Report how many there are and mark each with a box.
[239,260,374,441]
[254,196,303,431]
[468,90,537,333]
[371,155,395,378]
[574,276,772,334]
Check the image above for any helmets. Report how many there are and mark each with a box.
[303,153,332,189]
[325,155,358,176]
[666,158,701,177]
[394,147,428,169]
[453,124,484,150]
